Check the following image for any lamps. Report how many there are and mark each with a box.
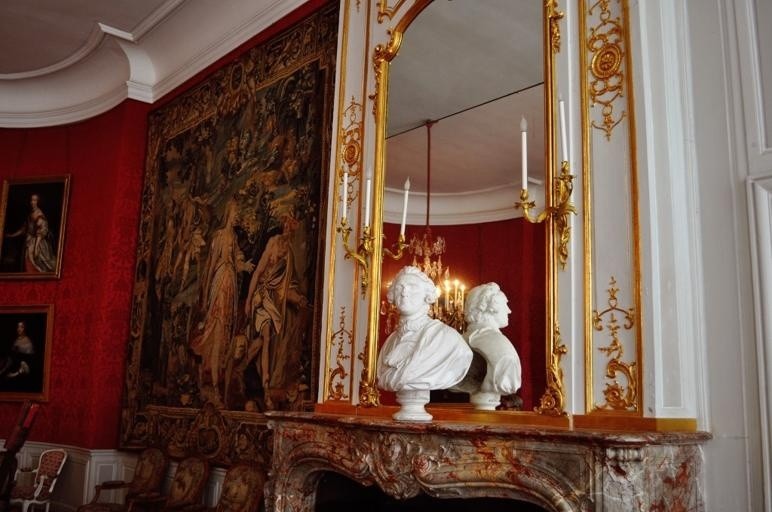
[336,164,376,300]
[381,119,466,335]
[513,92,577,271]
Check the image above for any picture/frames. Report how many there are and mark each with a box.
[0,174,71,280]
[0,304,55,403]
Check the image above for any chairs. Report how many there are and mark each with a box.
[9,448,67,512]
[78,446,265,511]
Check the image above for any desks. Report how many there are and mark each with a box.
[264,412,712,512]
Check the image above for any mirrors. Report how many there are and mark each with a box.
[358,0,564,416]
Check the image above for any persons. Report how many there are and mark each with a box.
[461,281,524,397]
[0,315,36,392]
[3,191,58,274]
[373,265,475,395]
